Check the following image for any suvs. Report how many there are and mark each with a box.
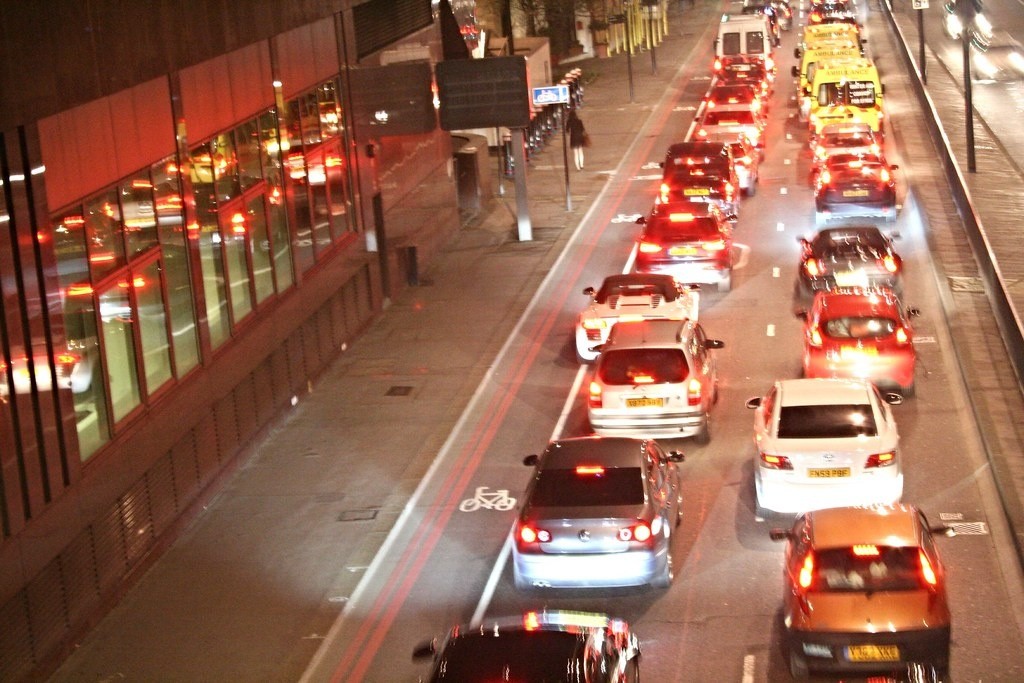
[769,501,954,683]
[632,200,732,293]
[584,323,726,448]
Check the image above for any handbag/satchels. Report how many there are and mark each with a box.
[582,129,592,148]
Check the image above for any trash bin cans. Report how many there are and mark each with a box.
[450,132,493,210]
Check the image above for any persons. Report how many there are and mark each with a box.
[566,111,585,170]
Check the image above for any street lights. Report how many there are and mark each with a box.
[365,107,392,306]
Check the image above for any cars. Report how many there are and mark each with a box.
[0,81,343,408]
[409,608,642,682]
[935,1,1023,82]
[649,0,791,219]
[574,273,704,368]
[791,0,904,219]
[795,224,907,312]
[797,286,919,405]
[512,434,687,595]
[748,377,902,536]
